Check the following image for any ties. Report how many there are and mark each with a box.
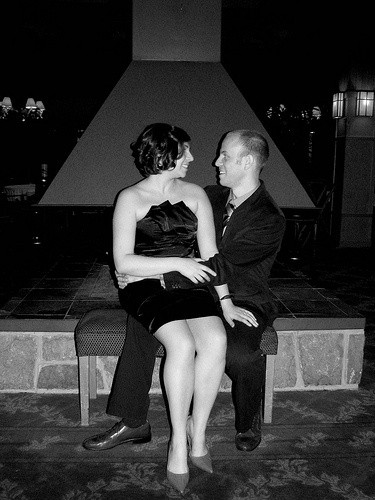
[221,201,236,238]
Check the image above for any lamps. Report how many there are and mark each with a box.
[0,96,45,121]
[332,93,345,118]
[267,101,321,122]
[356,89,375,117]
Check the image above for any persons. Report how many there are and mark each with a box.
[82,130,286,452]
[112,123,259,494]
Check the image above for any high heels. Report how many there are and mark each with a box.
[166,441,189,493]
[186,415,213,474]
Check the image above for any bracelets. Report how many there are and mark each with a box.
[219,295,234,301]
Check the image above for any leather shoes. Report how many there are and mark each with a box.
[236,410,261,451]
[83,420,151,450]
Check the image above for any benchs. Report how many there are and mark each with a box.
[74,309,278,427]
